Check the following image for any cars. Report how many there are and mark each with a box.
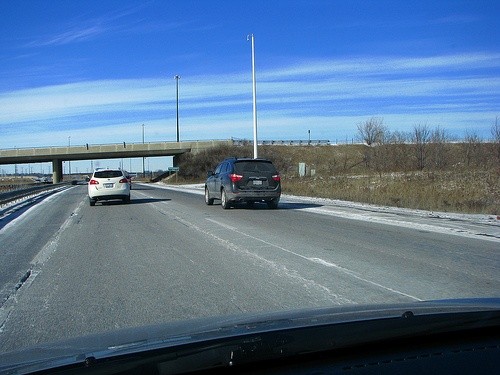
[87,167,129,205]
[204,158,283,208]
